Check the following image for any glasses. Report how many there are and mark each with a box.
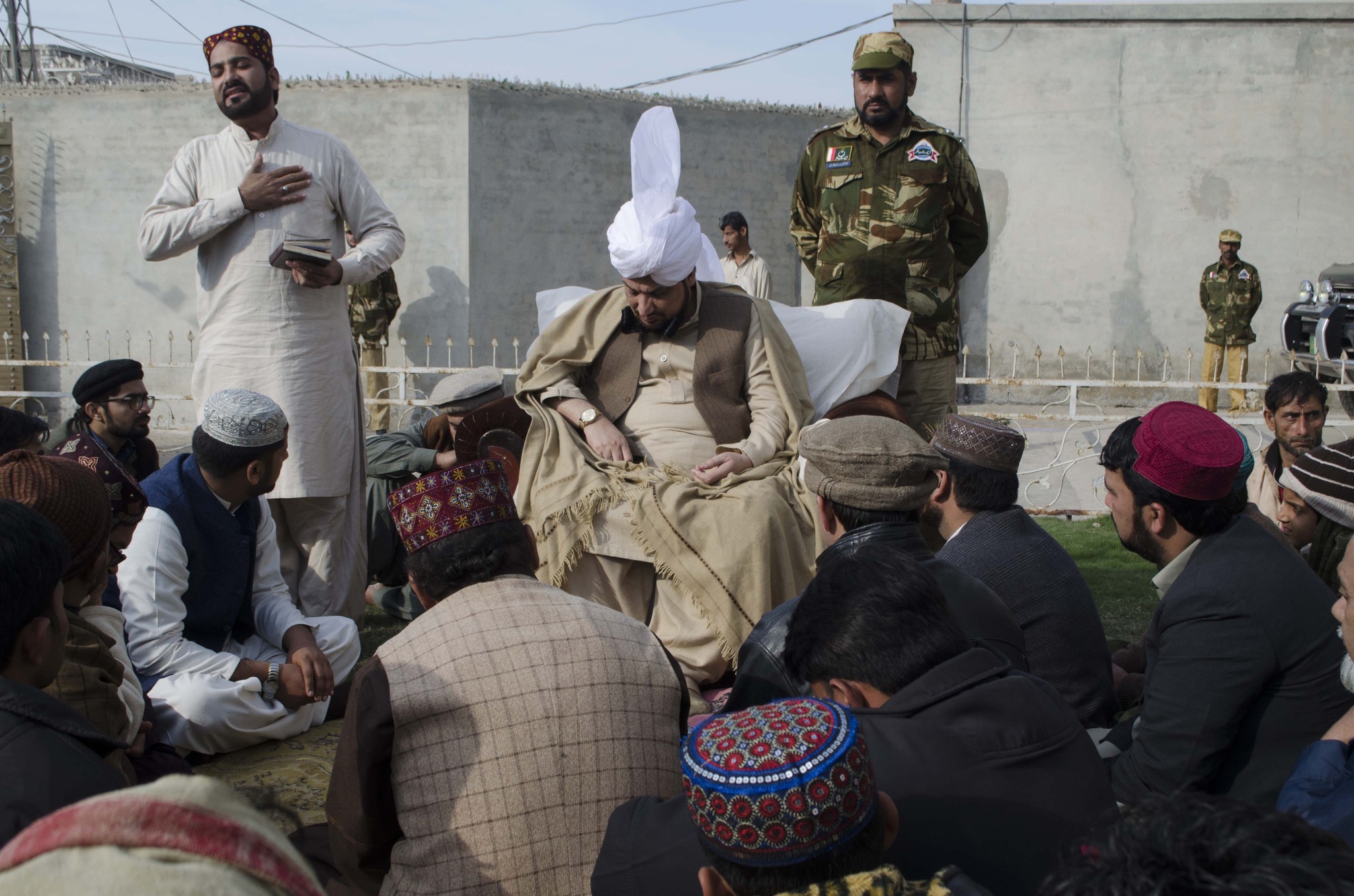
[100,395,156,410]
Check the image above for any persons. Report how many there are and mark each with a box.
[713,413,1029,715]
[1098,368,1354,853]
[506,104,822,719]
[0,351,508,895]
[789,30,991,443]
[137,23,405,631]
[591,534,1128,896]
[1199,229,1265,415]
[677,692,999,896]
[916,411,1124,730]
[335,455,692,896]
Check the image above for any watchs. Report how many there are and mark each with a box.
[579,407,605,432]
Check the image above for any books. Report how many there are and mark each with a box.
[268,238,333,271]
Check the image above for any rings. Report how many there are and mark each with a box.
[282,184,288,193]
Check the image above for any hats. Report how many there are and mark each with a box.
[200,389,288,447]
[605,104,702,288]
[1218,229,1242,243]
[933,412,1026,475]
[794,415,951,511]
[1277,438,1354,531]
[1132,400,1244,501]
[679,695,876,869]
[203,25,275,68]
[386,458,520,556]
[49,430,150,534]
[428,365,506,413]
[71,359,145,405]
[850,32,915,70]
[0,449,110,583]
